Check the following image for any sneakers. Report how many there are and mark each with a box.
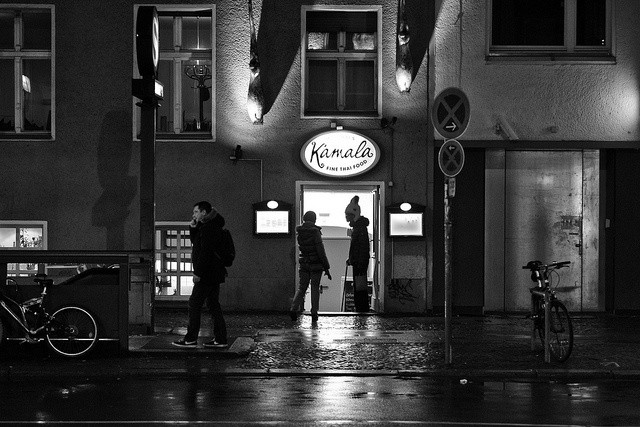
[203,339,228,347]
[172,339,198,347]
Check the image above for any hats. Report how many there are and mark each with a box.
[345,196,361,222]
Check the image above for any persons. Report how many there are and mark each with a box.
[171,200,231,348]
[288,211,332,323]
[345,195,370,313]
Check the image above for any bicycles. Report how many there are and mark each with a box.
[0,278,101,357]
[522,260,575,362]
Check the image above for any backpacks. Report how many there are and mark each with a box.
[214,228,236,267]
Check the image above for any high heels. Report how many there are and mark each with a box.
[311,311,319,322]
[289,311,297,321]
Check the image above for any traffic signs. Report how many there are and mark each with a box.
[438,138,466,178]
[432,88,471,139]
